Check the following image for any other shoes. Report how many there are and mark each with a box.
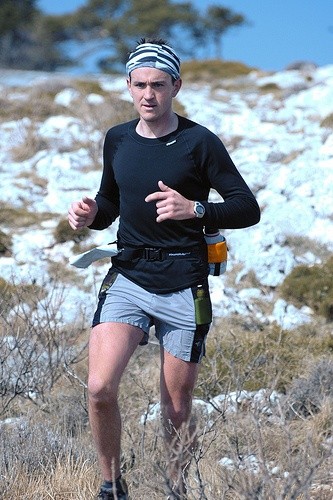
[97,477,128,500]
[166,486,187,500]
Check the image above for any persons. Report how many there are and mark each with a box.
[67,36,261,500]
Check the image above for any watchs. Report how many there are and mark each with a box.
[193,200,205,218]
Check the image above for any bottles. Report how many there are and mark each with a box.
[204,225,227,275]
